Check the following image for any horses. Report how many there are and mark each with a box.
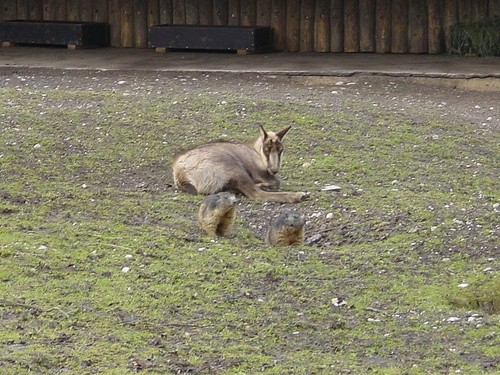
[172,122,308,203]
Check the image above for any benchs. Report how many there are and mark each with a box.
[0,20,110,51]
[149,23,272,56]
